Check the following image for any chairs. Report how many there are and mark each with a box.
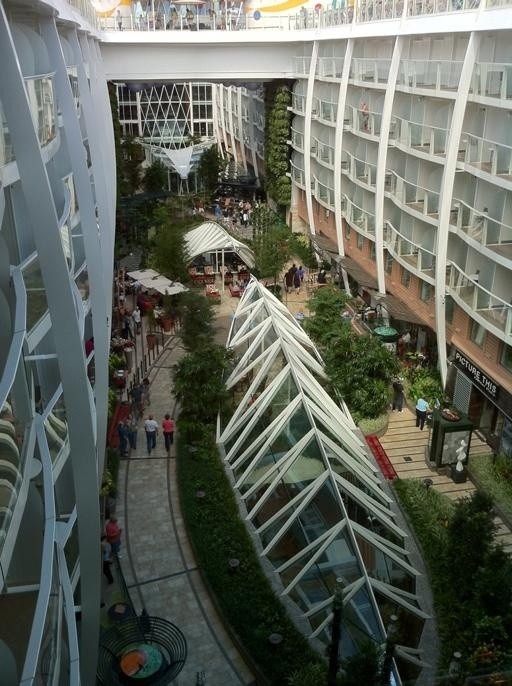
[187,262,250,300]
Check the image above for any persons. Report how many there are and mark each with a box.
[402,329,410,341]
[124,311,134,339]
[151,304,161,323]
[133,391,145,427]
[171,8,179,28]
[104,512,115,535]
[361,101,370,130]
[318,267,329,283]
[186,9,195,29]
[131,305,141,334]
[139,12,147,30]
[284,263,304,295]
[116,10,124,30]
[194,192,253,230]
[117,410,175,457]
[132,280,165,315]
[229,4,239,30]
[393,379,404,413]
[208,8,216,28]
[415,396,431,431]
[102,535,115,586]
[300,6,308,26]
[128,384,139,403]
[141,378,151,406]
[106,516,122,559]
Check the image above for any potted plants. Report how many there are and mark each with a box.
[146,288,172,349]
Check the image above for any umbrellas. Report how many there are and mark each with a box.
[173,0,205,5]
